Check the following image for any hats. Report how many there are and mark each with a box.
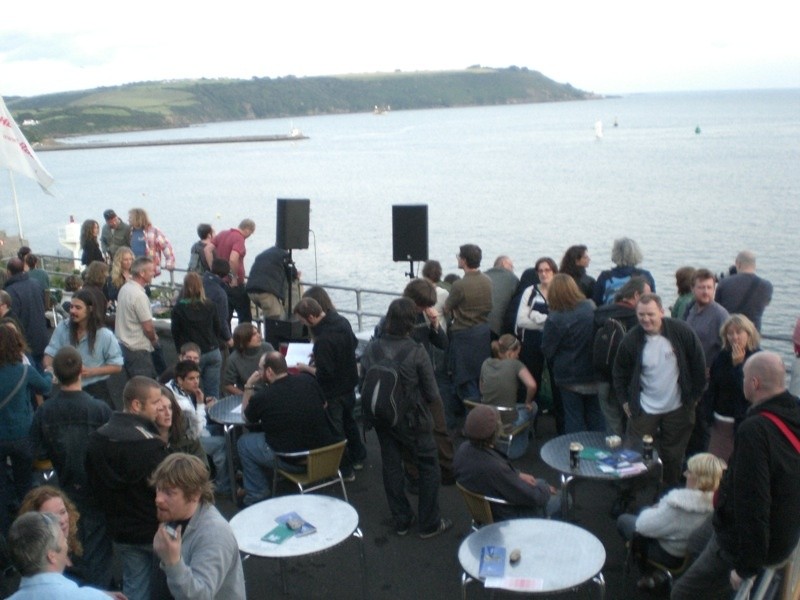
[465,404,500,437]
[211,260,233,276]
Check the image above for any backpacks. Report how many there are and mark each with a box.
[591,316,628,373]
[360,338,415,428]
[603,271,641,305]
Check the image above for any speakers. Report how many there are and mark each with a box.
[265,315,304,351]
[276,198,310,249]
[392,205,428,262]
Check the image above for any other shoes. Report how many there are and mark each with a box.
[354,460,364,469]
[611,491,635,518]
[397,514,417,534]
[418,518,455,538]
[343,472,356,482]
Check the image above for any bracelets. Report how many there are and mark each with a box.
[244,384,255,388]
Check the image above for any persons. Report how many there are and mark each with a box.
[715,251,772,331]
[374,244,573,519]
[358,297,454,539]
[617,453,725,591]
[561,245,596,298]
[594,236,656,304]
[708,314,760,458]
[483,256,519,341]
[0,206,362,599]
[594,278,651,440]
[684,269,730,361]
[671,351,800,599]
[613,294,706,485]
[512,257,557,415]
[543,275,604,432]
[671,266,695,319]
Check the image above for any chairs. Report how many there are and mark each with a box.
[463,396,536,457]
[273,439,349,503]
[455,480,551,531]
[622,532,693,600]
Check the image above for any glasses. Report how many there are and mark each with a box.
[456,254,462,258]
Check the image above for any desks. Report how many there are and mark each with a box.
[458,516,606,600]
[228,493,372,600]
[540,432,664,522]
[208,394,263,505]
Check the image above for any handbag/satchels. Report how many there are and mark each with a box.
[538,355,554,402]
[404,431,459,484]
[105,299,118,317]
[515,285,538,342]
[152,343,166,374]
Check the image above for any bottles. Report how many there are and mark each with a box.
[568,442,581,469]
[641,434,654,460]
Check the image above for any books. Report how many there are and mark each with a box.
[580,448,641,468]
[479,546,506,578]
[276,512,316,537]
[261,524,294,543]
